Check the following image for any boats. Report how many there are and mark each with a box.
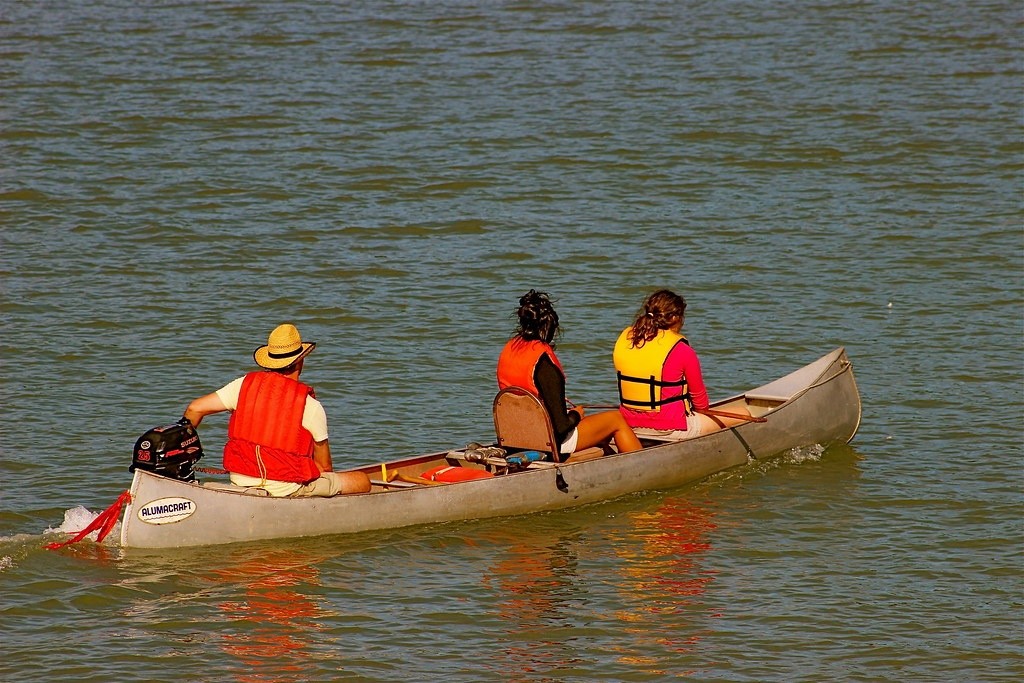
[121,346,862,547]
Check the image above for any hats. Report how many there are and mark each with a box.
[254,324,317,371]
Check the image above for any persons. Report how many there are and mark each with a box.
[495,289,644,453]
[613,289,752,439]
[181,323,372,500]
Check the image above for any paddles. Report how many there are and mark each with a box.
[580,403,768,424]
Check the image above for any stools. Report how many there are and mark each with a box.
[419,463,494,483]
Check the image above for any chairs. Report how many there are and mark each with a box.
[492,386,605,463]
